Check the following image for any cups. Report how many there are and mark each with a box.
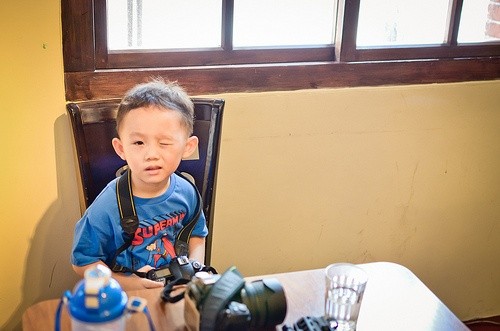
[324,262,367,331]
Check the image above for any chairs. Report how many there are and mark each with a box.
[65,96,224,276]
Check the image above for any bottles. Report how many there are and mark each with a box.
[53,265,156,331]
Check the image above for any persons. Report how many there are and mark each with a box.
[67,80,210,290]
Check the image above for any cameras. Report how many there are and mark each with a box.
[147,255,209,285]
[186,272,286,331]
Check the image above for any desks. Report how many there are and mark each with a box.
[20,260,470,331]
